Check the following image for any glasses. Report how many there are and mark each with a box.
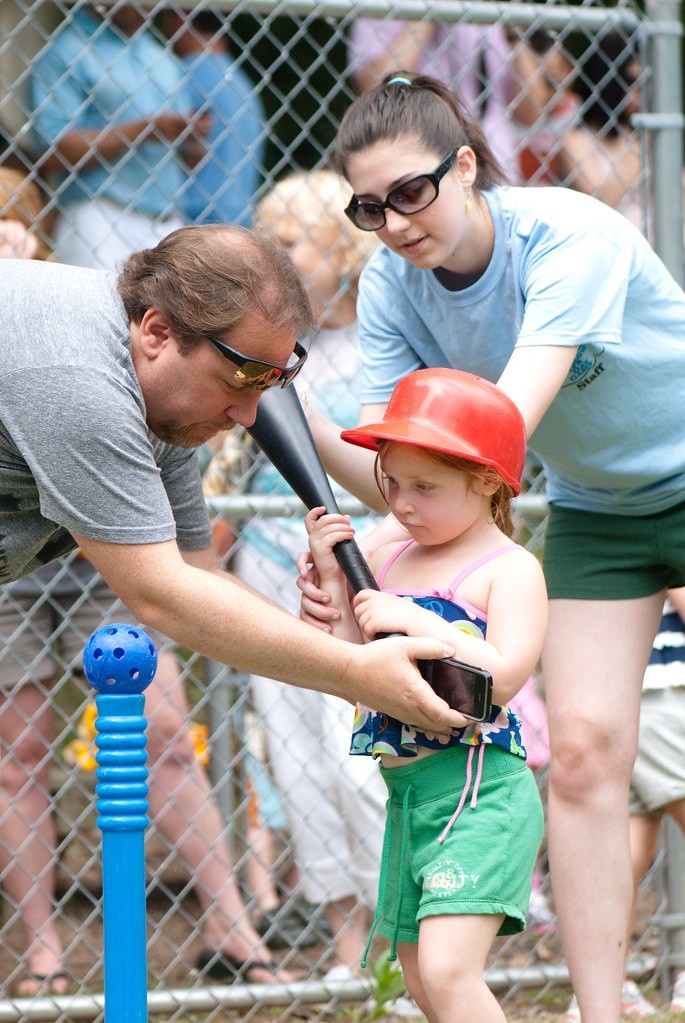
[346,148,459,231]
[204,333,308,390]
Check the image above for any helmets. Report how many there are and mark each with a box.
[340,367,525,496]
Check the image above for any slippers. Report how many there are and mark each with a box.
[199,952,289,988]
[18,973,69,995]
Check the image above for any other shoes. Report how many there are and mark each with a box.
[294,968,425,1018]
[671,973,685,1012]
[256,909,335,951]
[527,878,552,925]
[568,978,657,1016]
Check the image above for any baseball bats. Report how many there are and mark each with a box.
[248,371,408,644]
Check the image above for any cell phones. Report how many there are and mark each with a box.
[415,656,493,722]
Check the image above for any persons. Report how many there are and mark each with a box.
[563,595,685,1023]
[294,69,685,1023]
[0,223,474,734]
[348,15,519,185]
[163,8,265,230]
[0,550,294,998]
[303,368,550,1023]
[233,171,382,981]
[32,4,213,273]
[509,25,653,242]
